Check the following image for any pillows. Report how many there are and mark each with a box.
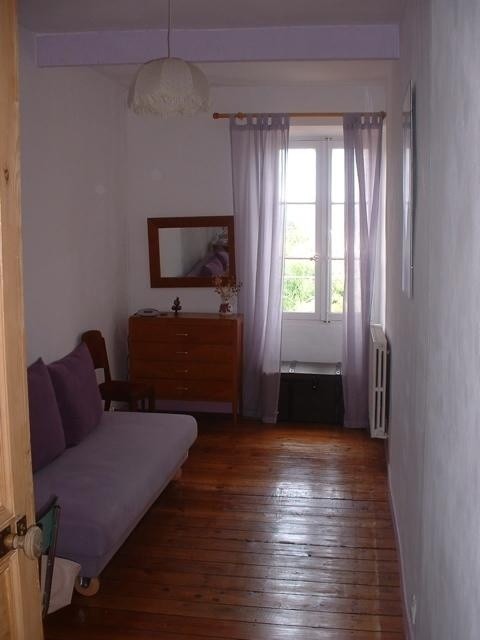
[47,343,103,447]
[215,251,229,269]
[200,257,222,278]
[28,358,66,472]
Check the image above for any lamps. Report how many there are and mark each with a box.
[130,0,212,117]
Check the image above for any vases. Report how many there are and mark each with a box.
[219,303,234,315]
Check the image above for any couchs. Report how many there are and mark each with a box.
[186,257,227,277]
[33,411,198,596]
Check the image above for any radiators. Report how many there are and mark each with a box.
[367,325,389,439]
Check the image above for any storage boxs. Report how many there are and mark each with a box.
[278,361,344,425]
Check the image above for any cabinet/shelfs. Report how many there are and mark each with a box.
[128,312,244,425]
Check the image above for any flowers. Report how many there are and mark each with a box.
[212,275,243,303]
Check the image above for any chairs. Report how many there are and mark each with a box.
[81,330,155,412]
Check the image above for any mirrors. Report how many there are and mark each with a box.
[147,216,234,288]
